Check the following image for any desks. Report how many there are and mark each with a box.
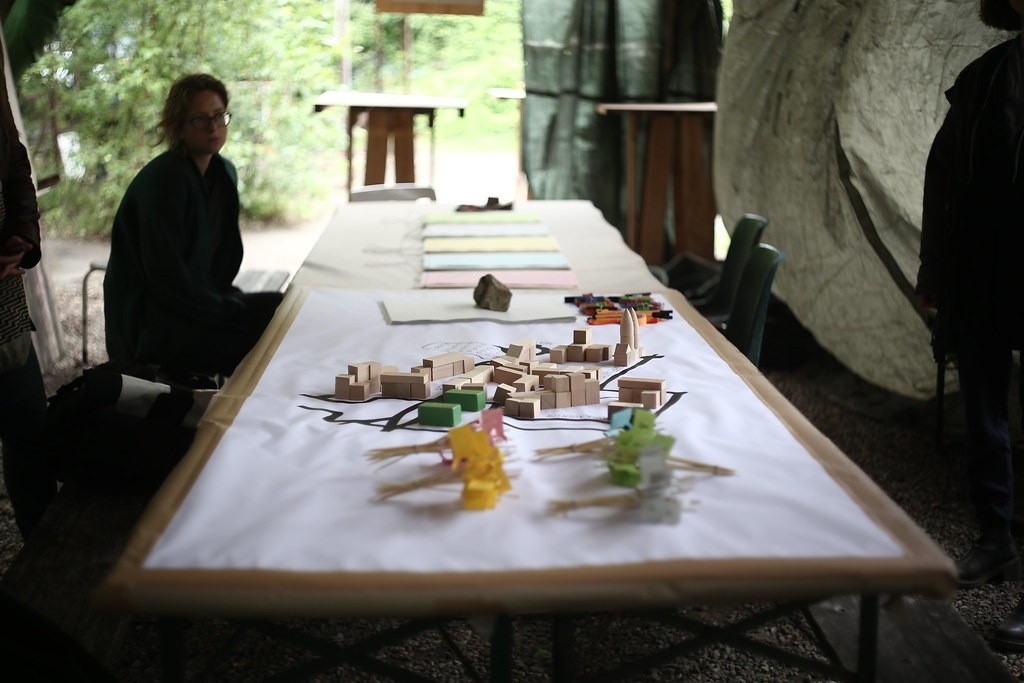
[88,194,960,683]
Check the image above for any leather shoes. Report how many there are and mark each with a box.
[994,593,1024,650]
[951,536,1024,589]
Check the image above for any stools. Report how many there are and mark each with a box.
[797,591,1014,683]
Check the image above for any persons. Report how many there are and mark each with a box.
[917,1,1023,649]
[103,73,285,390]
[0,98,56,542]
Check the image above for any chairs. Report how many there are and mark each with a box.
[716,243,782,368]
[82,263,108,365]
[680,215,769,325]
[646,249,725,299]
[101,279,223,393]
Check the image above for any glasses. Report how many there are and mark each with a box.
[184,111,232,132]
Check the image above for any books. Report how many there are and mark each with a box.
[420,209,580,291]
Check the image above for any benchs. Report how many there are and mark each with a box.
[0,414,198,673]
